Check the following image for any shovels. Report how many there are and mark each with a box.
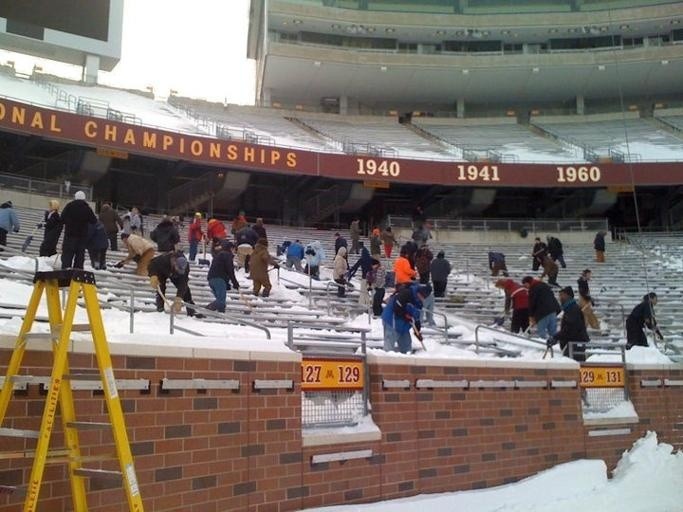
[21,217,45,252]
[198,239,210,268]
[246,268,275,279]
[114,260,130,268]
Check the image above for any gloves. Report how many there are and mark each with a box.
[415,320,421,334]
[402,312,412,321]
[150,276,158,288]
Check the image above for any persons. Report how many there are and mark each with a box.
[381,284,433,354]
[285,218,451,326]
[35,190,280,319]
[0,200,20,257]
[488,236,600,361]
[623,293,663,351]
[593,231,607,262]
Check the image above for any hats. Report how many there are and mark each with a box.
[418,284,432,298]
[75,191,85,200]
[560,286,574,297]
[175,258,188,275]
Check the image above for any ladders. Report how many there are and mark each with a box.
[0,270,144,512]
